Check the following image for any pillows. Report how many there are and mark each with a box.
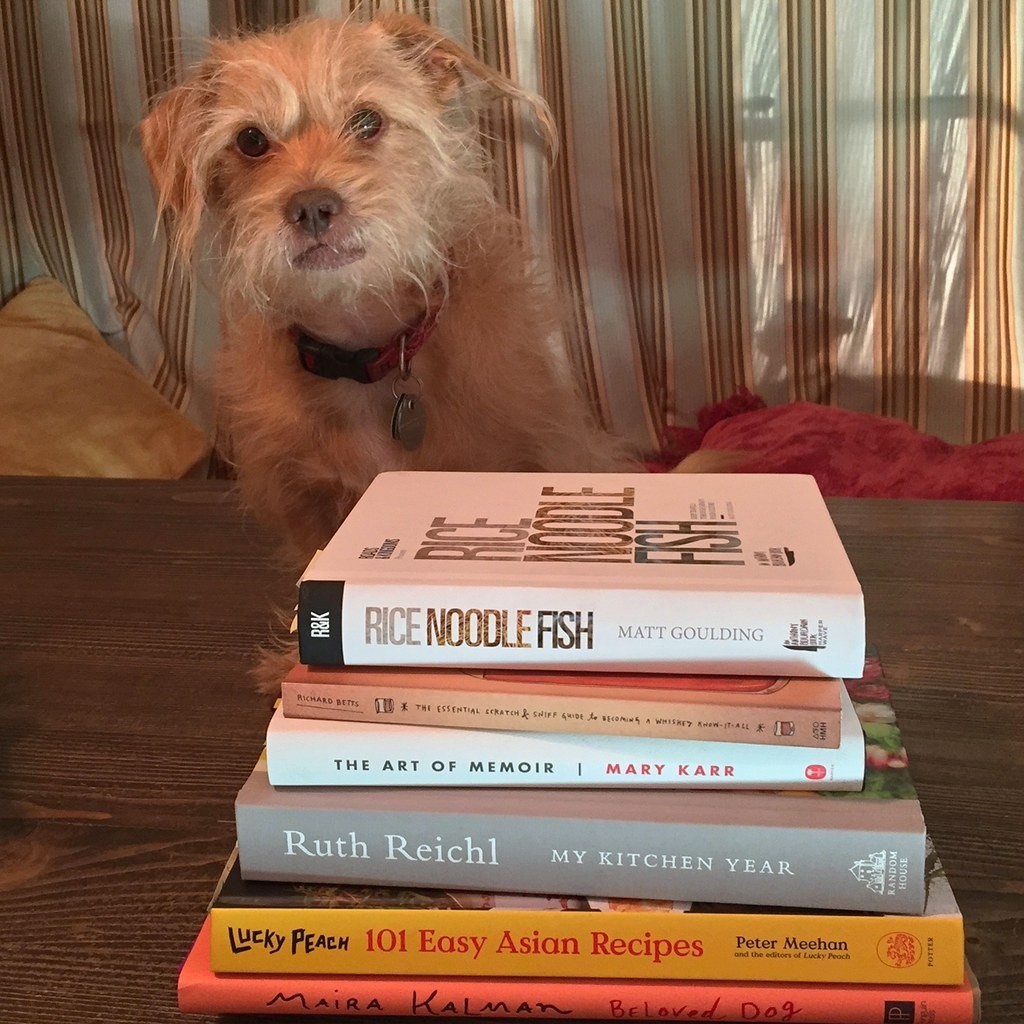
[0,276,212,481]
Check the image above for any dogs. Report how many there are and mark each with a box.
[138,13,656,695]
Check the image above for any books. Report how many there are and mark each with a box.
[177,471,983,1024]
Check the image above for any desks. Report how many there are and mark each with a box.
[0,474,1024,1024]
